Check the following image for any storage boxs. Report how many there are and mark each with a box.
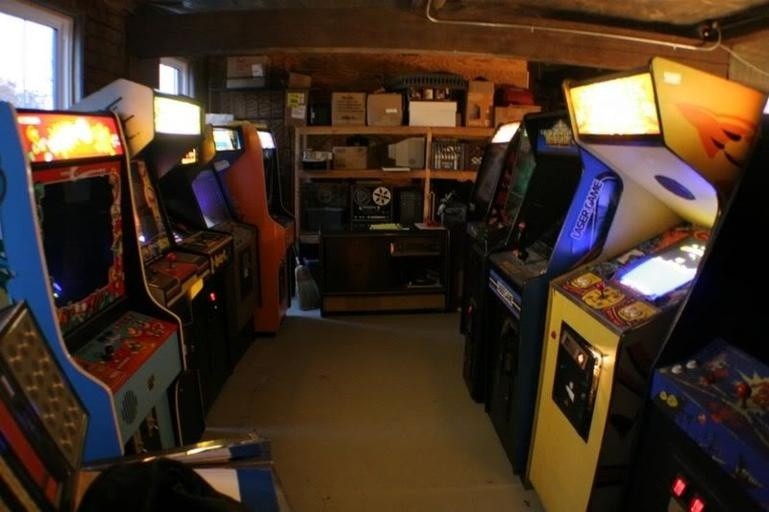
[464,79,494,127]
[331,92,365,125]
[287,72,312,89]
[332,147,367,170]
[284,89,308,126]
[495,105,541,127]
[367,94,402,125]
[227,55,270,89]
[408,102,456,126]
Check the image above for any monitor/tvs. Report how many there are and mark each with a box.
[130,165,163,249]
[616,231,717,303]
[34,174,126,336]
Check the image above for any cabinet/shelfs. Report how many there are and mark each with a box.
[293,127,499,318]
[208,77,291,210]
